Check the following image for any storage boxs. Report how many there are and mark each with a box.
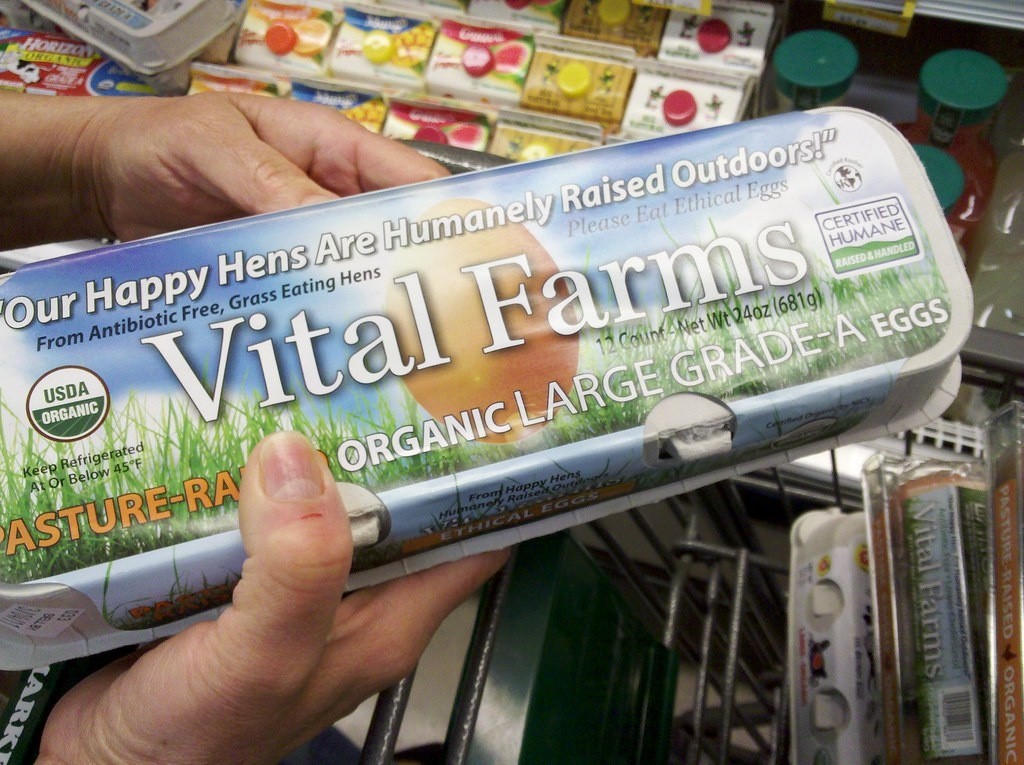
[786,401,1024,765]
[0,0,782,163]
[0,106,973,670]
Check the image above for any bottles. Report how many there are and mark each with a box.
[758,31,1023,340]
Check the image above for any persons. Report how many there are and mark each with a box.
[0,88,512,765]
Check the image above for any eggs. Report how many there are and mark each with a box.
[379,198,578,444]
